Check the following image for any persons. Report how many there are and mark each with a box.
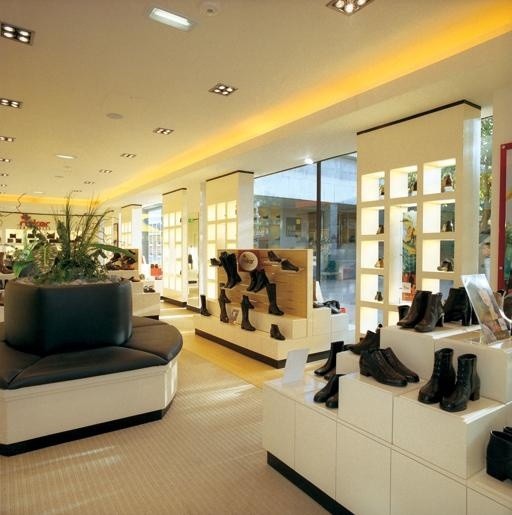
[467,277,510,340]
[482,242,490,283]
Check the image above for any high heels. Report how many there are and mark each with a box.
[399,283,484,332]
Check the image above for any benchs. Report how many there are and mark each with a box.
[1,272,182,454]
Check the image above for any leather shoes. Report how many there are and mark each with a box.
[359,345,419,387]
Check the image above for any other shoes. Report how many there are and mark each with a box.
[313,300,341,314]
[375,292,382,301]
[442,220,453,232]
[441,175,452,187]
[375,258,384,268]
[380,185,384,195]
[437,261,452,271]
[413,181,418,191]
[377,225,383,234]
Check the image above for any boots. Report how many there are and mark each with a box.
[313,341,349,408]
[402,272,416,288]
[102,248,165,294]
[349,324,383,355]
[402,220,415,245]
[418,348,480,412]
[486,420,511,484]
[185,250,298,340]
[0,252,71,308]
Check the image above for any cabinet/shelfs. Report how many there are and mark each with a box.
[162,188,189,305]
[264,321,512,514]
[120,205,142,265]
[197,249,348,367]
[354,103,478,332]
[115,270,161,319]
[204,173,254,256]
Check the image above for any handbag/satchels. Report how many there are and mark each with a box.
[6,233,58,243]
